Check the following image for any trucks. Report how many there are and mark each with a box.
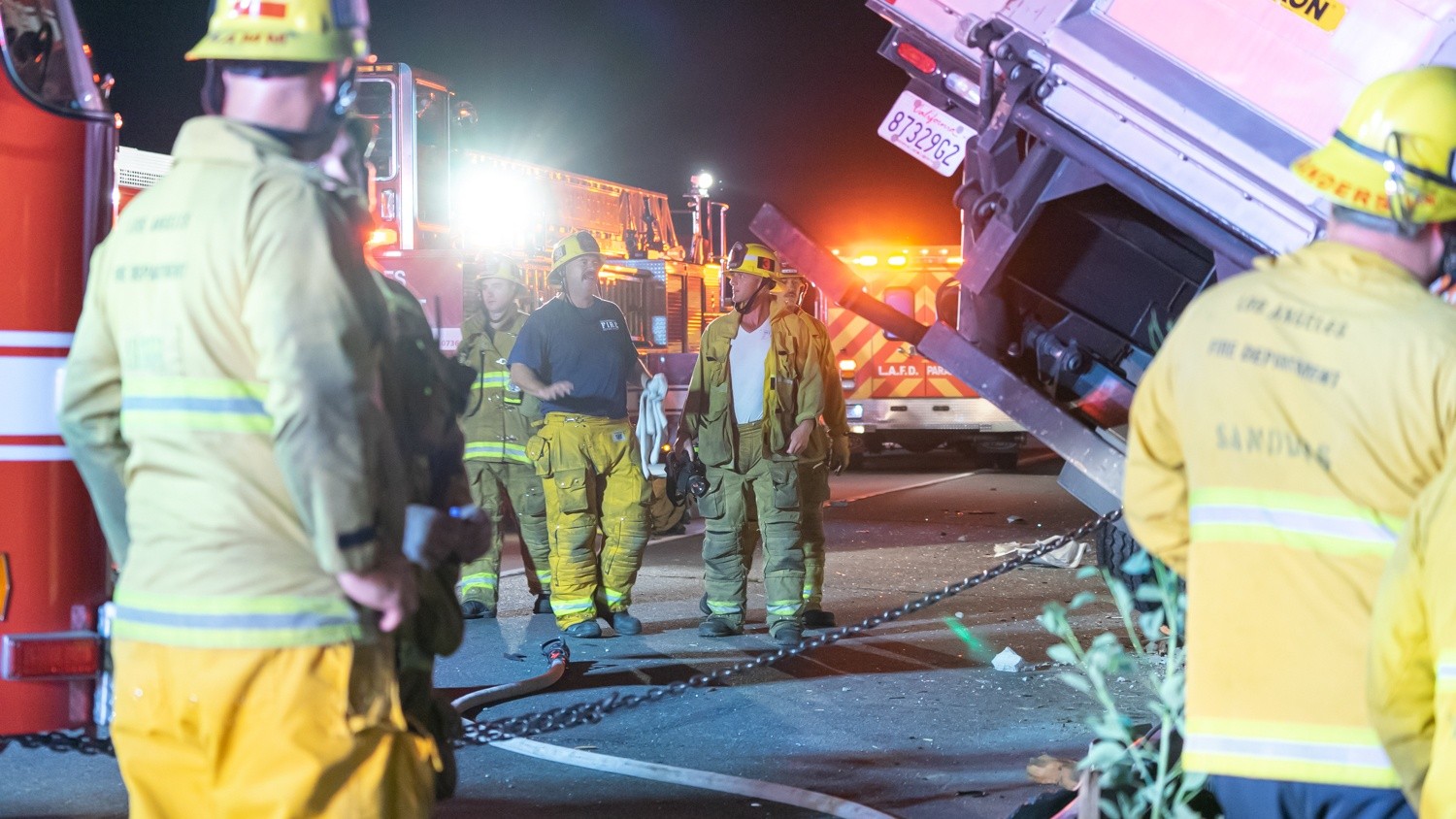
[748,1,1456,614]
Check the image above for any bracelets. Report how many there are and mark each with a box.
[645,378,652,388]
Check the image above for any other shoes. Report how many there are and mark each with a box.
[533,593,554,613]
[566,619,602,637]
[460,600,496,618]
[700,591,712,615]
[776,628,802,645]
[597,609,642,635]
[801,609,834,626]
[698,620,744,636]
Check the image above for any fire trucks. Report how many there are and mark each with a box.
[1,0,1032,735]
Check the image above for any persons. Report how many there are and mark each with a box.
[312,100,491,799]
[54,0,444,818]
[521,269,668,636]
[1121,61,1455,819]
[453,252,552,618]
[668,242,850,646]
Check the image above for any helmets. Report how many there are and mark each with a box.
[314,101,369,209]
[475,254,528,295]
[545,231,607,285]
[772,251,806,279]
[1291,64,1456,224]
[721,243,787,293]
[184,0,370,63]
